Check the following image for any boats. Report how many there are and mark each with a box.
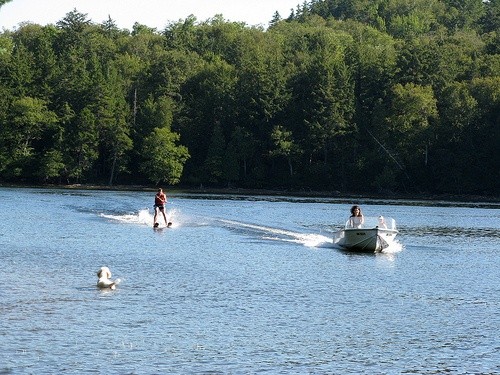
[331,215,399,253]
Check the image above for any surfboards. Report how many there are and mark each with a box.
[156,226,168,230]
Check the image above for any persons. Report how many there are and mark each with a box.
[377,216,387,229]
[153,188,172,228]
[349,206,364,230]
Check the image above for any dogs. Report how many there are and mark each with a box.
[96,266,121,289]
